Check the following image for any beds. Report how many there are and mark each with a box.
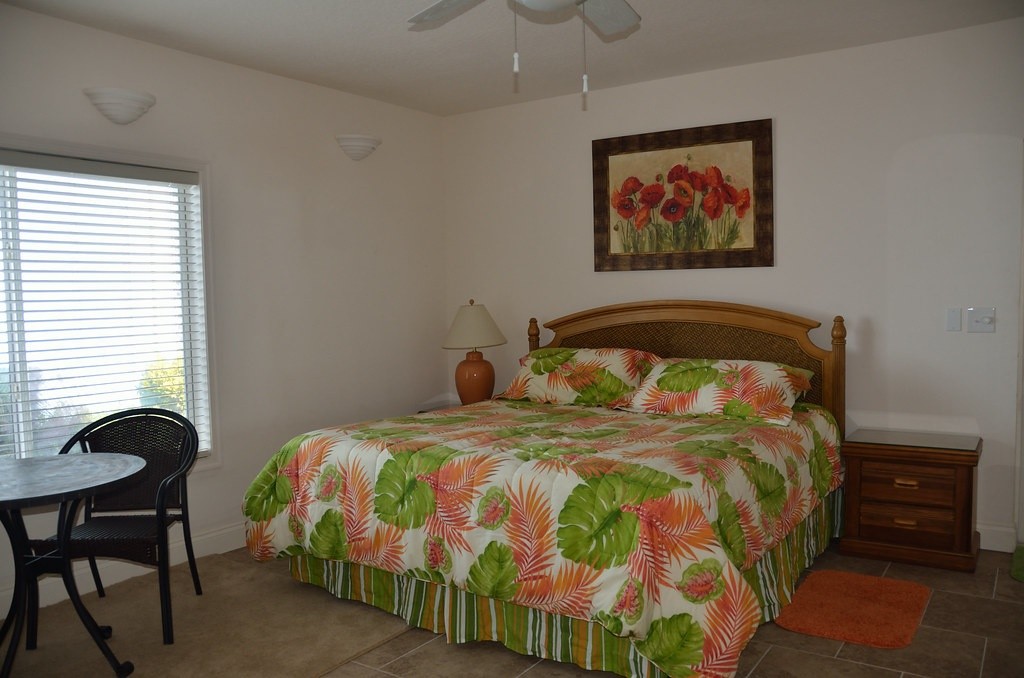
[241,300,848,678]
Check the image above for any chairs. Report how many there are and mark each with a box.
[27,408,200,648]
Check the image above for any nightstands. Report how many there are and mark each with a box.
[840,428,983,573]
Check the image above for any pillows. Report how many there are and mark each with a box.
[494,347,660,409]
[619,357,816,426]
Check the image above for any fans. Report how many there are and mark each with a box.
[408,0,641,36]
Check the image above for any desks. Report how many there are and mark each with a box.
[0,452,147,678]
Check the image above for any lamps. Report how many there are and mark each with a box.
[441,299,509,404]
[513,0,589,93]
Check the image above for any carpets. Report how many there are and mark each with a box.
[775,568,932,649]
[0,553,415,678]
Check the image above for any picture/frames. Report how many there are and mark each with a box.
[591,118,773,272]
